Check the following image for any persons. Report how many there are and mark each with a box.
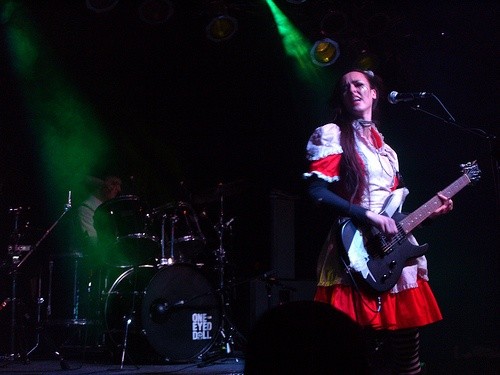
[303,70,455,375]
[78,170,123,245]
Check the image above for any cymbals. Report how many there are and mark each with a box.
[208,180,235,194]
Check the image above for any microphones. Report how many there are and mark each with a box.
[388,90,434,103]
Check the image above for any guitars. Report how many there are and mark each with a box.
[333,158,483,294]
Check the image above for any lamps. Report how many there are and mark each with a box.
[86,0,238,41]
[309,26,340,67]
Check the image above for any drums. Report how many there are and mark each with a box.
[104,262,224,362]
[93,195,162,268]
[151,201,207,260]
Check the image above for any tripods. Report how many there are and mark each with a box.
[196,196,250,367]
